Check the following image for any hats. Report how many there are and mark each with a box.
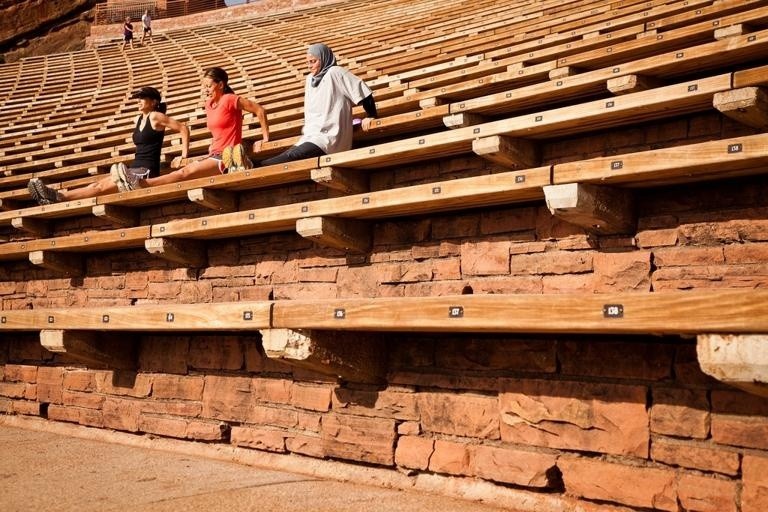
[131,87,161,101]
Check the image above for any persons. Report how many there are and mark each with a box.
[111,67,269,192]
[141,10,153,46]
[221,44,377,173]
[28,87,191,207]
[121,17,135,53]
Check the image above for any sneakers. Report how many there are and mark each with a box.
[110,163,128,193]
[27,179,45,206]
[222,146,236,174]
[232,143,253,171]
[33,177,59,205]
[118,161,143,191]
